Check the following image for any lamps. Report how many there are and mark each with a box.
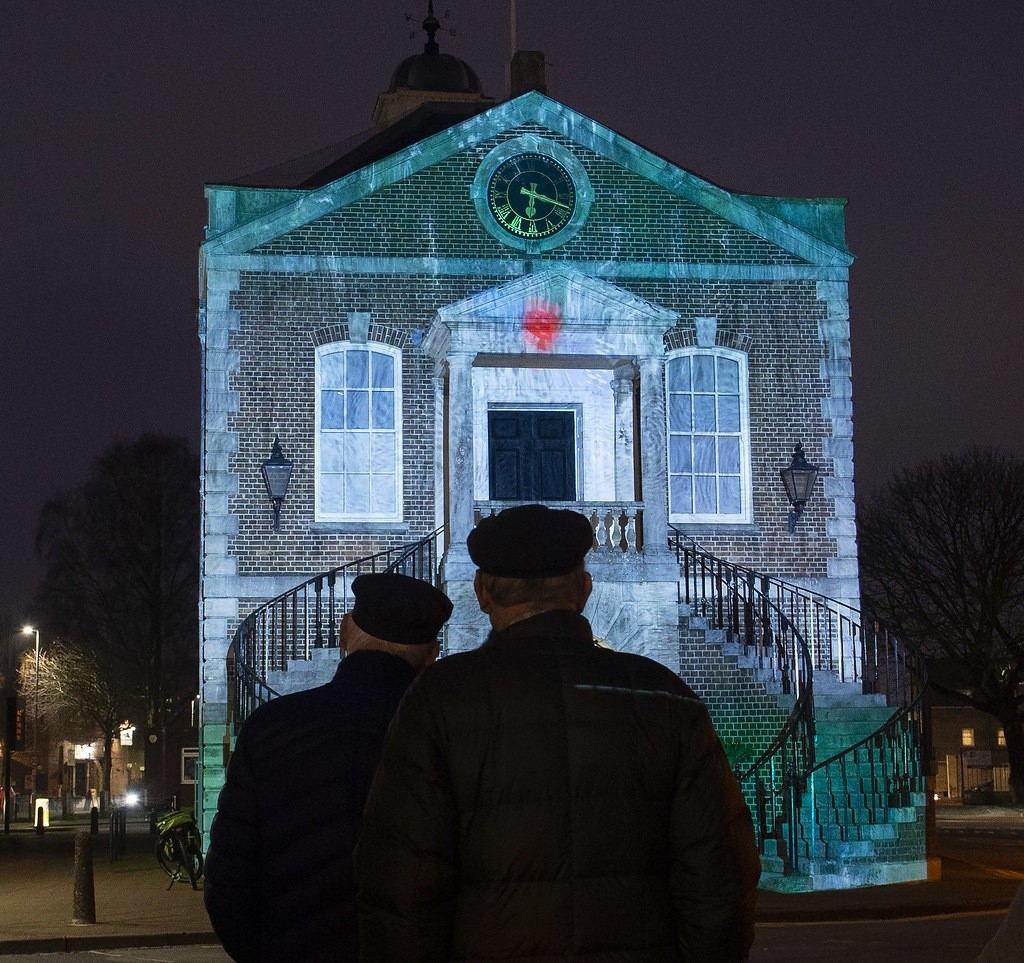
[781,440,819,534]
[261,435,294,532]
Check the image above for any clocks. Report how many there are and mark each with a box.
[490,154,575,238]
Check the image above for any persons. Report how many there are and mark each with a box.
[202,571,454,963]
[354,506,763,963]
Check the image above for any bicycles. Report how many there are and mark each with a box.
[145,787,204,892]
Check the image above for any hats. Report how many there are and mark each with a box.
[351,572,455,646]
[467,504,595,579]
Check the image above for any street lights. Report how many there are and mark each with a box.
[19,626,39,824]
